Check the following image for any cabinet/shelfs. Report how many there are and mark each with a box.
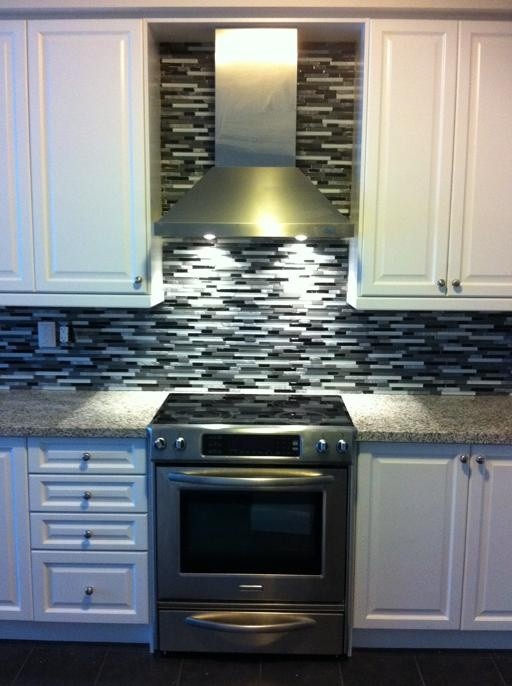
[0,435,158,661]
[347,443,510,655]
[344,18,509,315]
[0,12,168,311]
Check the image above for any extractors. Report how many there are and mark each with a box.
[153,26,355,245]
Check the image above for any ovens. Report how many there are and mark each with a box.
[154,469,346,657]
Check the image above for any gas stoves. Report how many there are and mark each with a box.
[148,392,355,468]
